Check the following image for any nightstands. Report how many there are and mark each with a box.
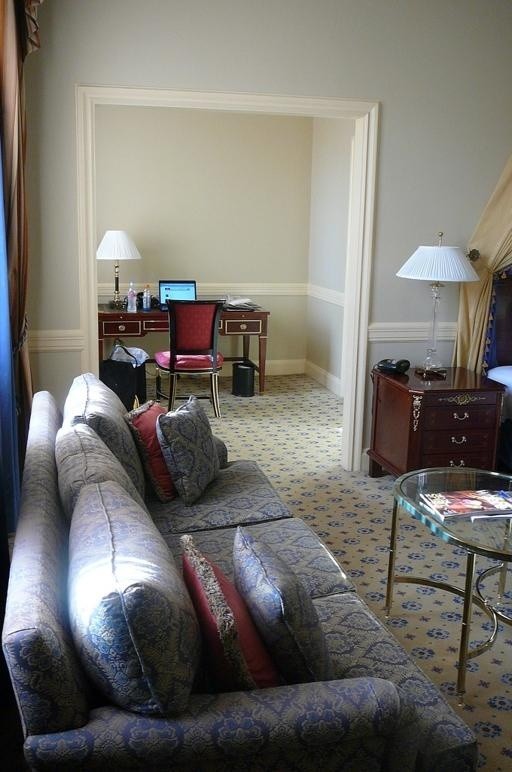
[366,360,509,505]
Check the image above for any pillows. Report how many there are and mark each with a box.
[47,425,152,526]
[57,370,147,505]
[231,518,333,681]
[172,522,283,692]
[121,393,175,508]
[58,474,206,722]
[152,389,223,504]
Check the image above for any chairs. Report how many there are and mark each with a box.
[152,295,225,420]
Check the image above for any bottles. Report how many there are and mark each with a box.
[142,282,151,311]
[127,280,136,312]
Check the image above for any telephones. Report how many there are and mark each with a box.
[378,359,410,373]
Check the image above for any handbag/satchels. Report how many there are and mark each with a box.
[102,338,148,405]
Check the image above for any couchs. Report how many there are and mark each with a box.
[1,385,484,771]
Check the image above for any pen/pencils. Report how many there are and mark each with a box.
[419,501,435,515]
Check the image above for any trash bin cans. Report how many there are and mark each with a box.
[231,363,256,397]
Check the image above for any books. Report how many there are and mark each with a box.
[418,488,512,522]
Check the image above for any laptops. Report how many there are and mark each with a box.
[159,280,196,311]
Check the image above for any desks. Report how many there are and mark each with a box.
[98,299,272,397]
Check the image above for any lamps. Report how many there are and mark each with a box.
[96,227,141,309]
[394,231,484,383]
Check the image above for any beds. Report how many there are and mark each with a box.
[479,254,511,494]
